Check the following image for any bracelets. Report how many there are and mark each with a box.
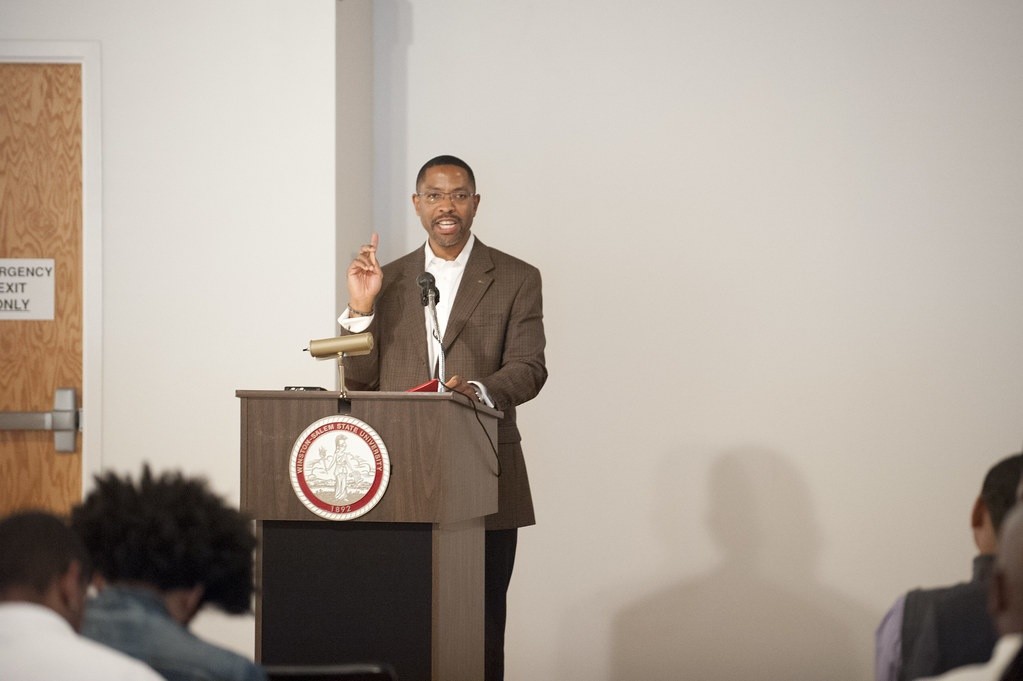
[346,300,376,317]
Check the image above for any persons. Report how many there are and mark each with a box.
[868,450,1023,681]
[0,458,269,681]
[337,156,548,681]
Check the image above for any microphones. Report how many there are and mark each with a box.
[417,272,436,295]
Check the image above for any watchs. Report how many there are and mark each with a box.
[469,385,485,405]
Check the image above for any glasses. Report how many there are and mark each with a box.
[418,191,474,203]
